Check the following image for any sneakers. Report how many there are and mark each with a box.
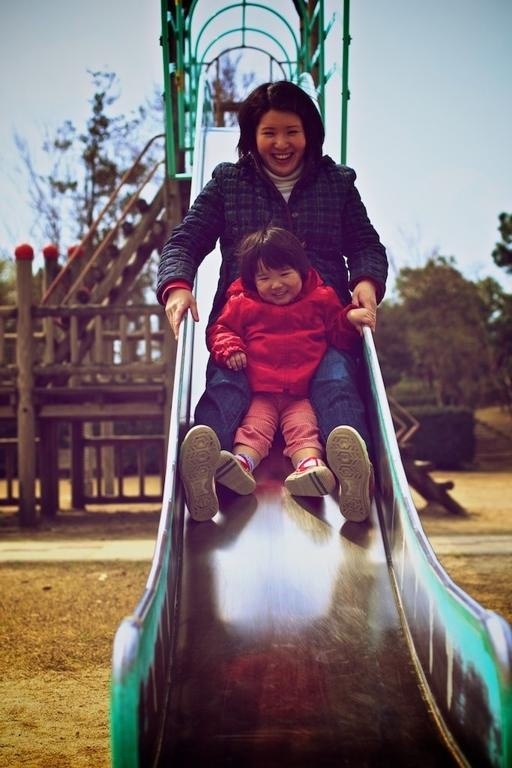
[178,425,219,522]
[284,457,336,497]
[326,425,375,523]
[214,449,257,496]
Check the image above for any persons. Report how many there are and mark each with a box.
[205,225,376,503]
[153,79,391,526]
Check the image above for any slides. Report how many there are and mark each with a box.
[108,72,512,768]
